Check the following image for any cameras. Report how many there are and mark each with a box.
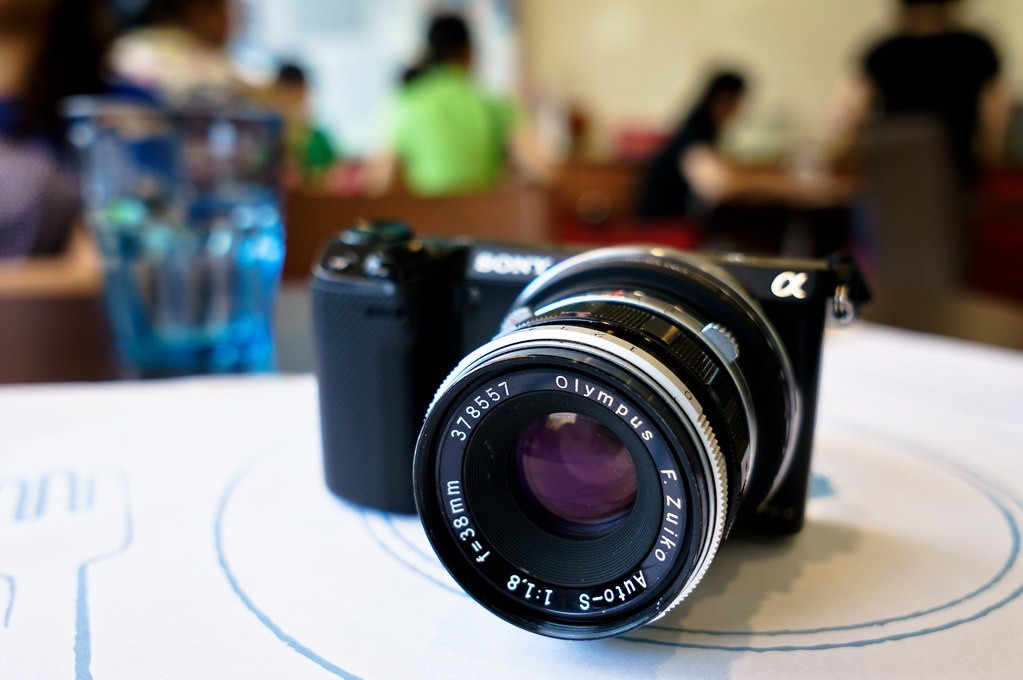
[307,214,830,643]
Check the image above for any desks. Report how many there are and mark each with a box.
[0,324,1023,680]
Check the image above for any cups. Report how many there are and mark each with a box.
[61,102,287,378]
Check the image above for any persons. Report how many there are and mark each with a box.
[274,64,332,169]
[857,0,1007,192]
[106,0,228,87]
[396,15,512,198]
[634,71,742,223]
